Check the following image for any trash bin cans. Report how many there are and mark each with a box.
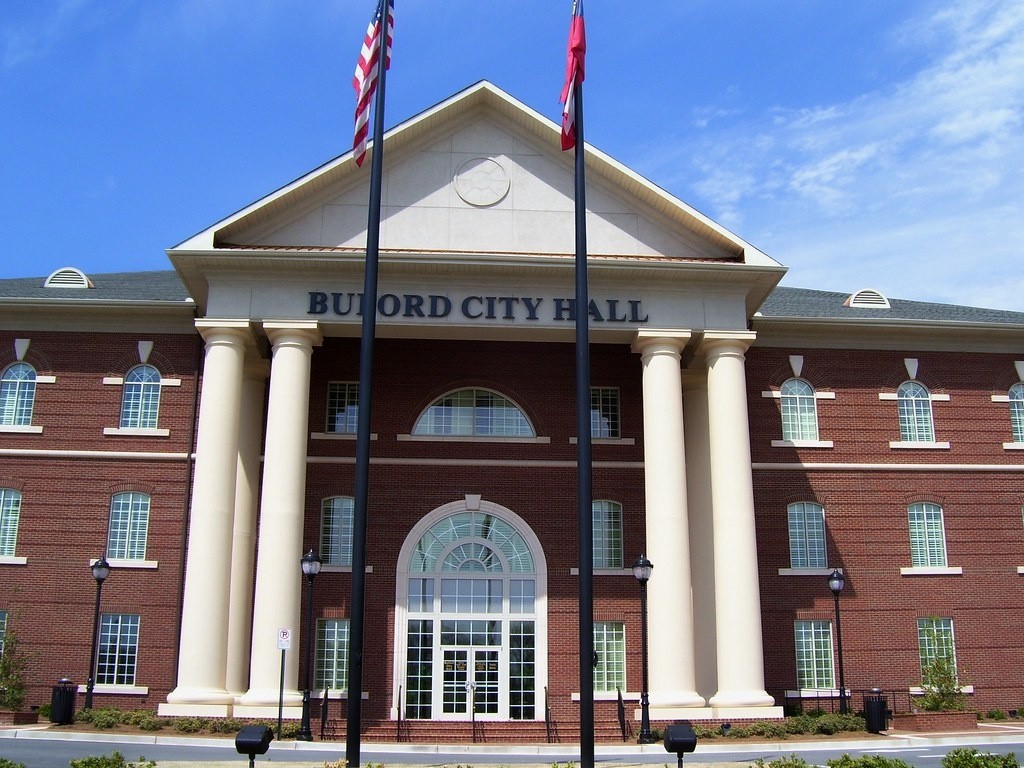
[861,688,891,733]
[49,678,79,724]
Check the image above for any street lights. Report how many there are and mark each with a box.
[631,554,656,745]
[82,556,111,712]
[826,567,850,716]
[294,547,323,741]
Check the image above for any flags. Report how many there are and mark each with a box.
[352,0,395,168]
[558,0,586,151]
